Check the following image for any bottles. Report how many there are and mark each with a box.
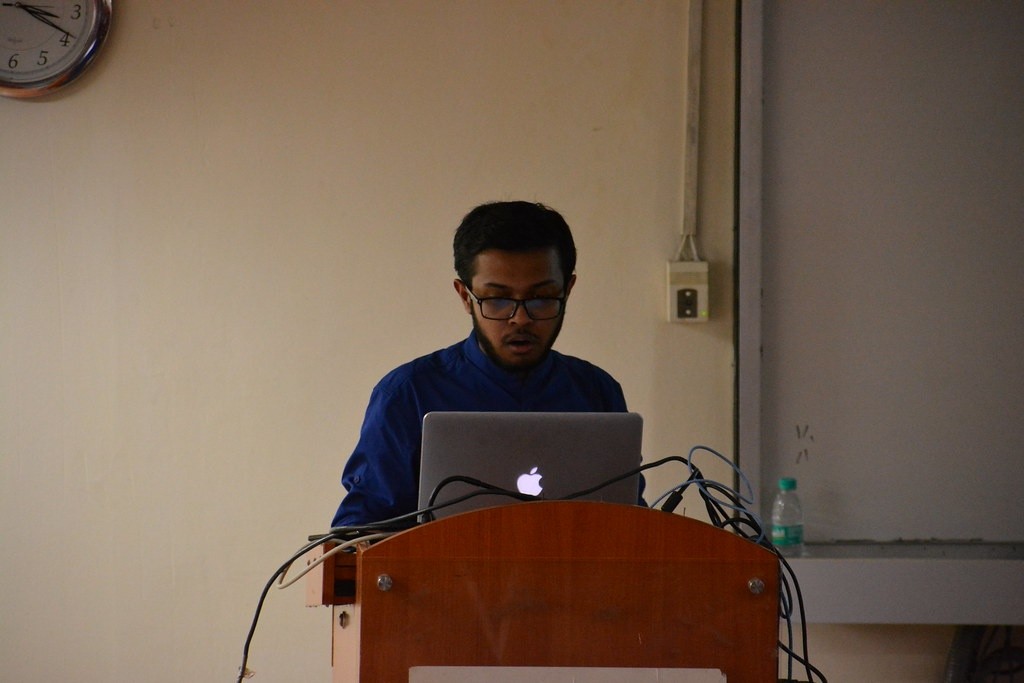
[772,479,803,556]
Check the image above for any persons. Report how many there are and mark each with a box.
[331,201,649,543]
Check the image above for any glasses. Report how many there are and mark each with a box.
[464,285,567,320]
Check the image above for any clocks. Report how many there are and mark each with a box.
[0,0,112,99]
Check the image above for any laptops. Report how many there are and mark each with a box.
[416,411,644,525]
